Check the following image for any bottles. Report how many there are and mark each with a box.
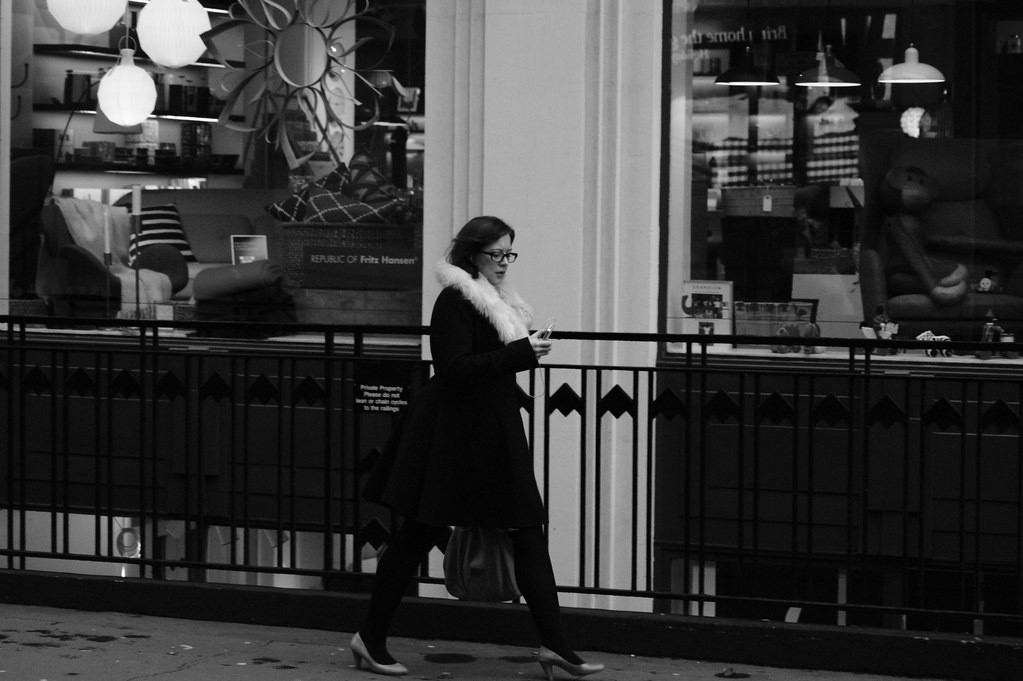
[109,10,149,58]
[354,70,399,123]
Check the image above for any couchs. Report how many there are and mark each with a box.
[853,110,1023,347]
[10,156,423,343]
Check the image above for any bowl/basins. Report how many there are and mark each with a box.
[82,121,213,175]
[211,154,240,175]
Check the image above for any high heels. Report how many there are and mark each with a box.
[538,646,604,680]
[350,633,407,673]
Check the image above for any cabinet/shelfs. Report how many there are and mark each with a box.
[32,0,240,174]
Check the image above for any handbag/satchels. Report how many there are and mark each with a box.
[443,522,523,602]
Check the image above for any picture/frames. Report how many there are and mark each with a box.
[685,278,734,349]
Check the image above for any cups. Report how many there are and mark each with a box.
[64,74,226,119]
[74,146,91,165]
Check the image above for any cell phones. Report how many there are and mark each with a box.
[536,324,554,360]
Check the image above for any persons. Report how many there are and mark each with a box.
[792,185,843,250]
[349,217,605,681]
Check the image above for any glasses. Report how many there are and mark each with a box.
[478,249,518,264]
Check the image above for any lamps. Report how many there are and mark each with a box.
[47,0,212,125]
[714,0,946,87]
[50,109,207,327]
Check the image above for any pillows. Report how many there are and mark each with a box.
[265,163,352,221]
[122,202,197,263]
[349,145,400,205]
[305,178,402,223]
